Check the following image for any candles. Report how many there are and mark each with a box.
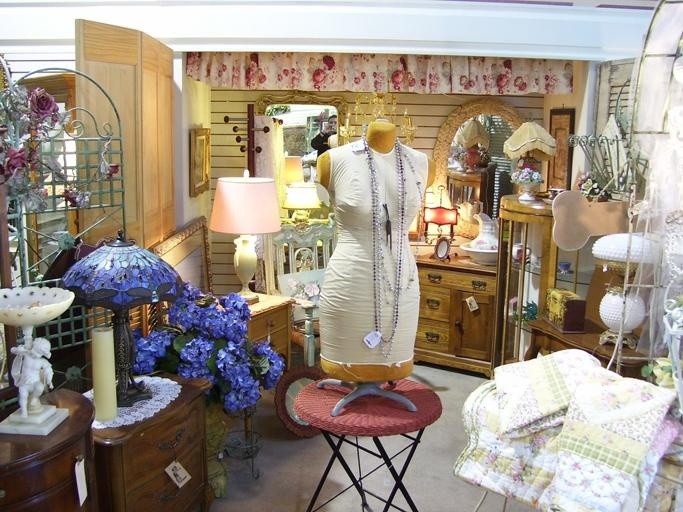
[91,327,117,420]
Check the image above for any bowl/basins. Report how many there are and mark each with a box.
[459,242,499,265]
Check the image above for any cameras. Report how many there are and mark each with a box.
[328,124,336,132]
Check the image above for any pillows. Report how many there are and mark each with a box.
[278,267,326,323]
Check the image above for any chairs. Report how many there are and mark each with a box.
[262,214,338,350]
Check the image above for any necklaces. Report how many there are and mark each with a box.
[362,128,425,360]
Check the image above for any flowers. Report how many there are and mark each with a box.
[577,171,609,198]
[134,284,287,419]
[0,87,120,212]
[511,160,545,185]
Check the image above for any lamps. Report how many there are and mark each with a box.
[423,205,458,262]
[592,233,662,350]
[286,184,322,222]
[205,170,283,305]
[339,93,418,147]
[503,121,558,193]
[458,119,490,171]
[63,231,185,406]
[283,156,304,184]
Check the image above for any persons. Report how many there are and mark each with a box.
[311,114,337,155]
[314,119,436,382]
[11,338,56,418]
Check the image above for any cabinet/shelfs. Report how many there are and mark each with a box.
[82,371,213,512]
[448,160,496,221]
[414,253,504,379]
[0,387,97,512]
[490,193,601,378]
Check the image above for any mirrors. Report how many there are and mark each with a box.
[275,368,327,438]
[433,100,524,239]
[258,89,350,184]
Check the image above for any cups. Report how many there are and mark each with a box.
[511,242,532,262]
[547,188,567,201]
[557,261,571,272]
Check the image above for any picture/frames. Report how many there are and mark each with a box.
[189,127,211,197]
[547,108,574,192]
[150,216,214,300]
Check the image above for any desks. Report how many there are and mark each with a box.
[295,381,442,512]
[525,318,654,379]
[222,292,296,442]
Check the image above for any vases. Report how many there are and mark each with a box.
[202,390,229,500]
[520,183,535,201]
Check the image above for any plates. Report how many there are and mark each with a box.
[542,198,553,205]
[433,236,450,261]
